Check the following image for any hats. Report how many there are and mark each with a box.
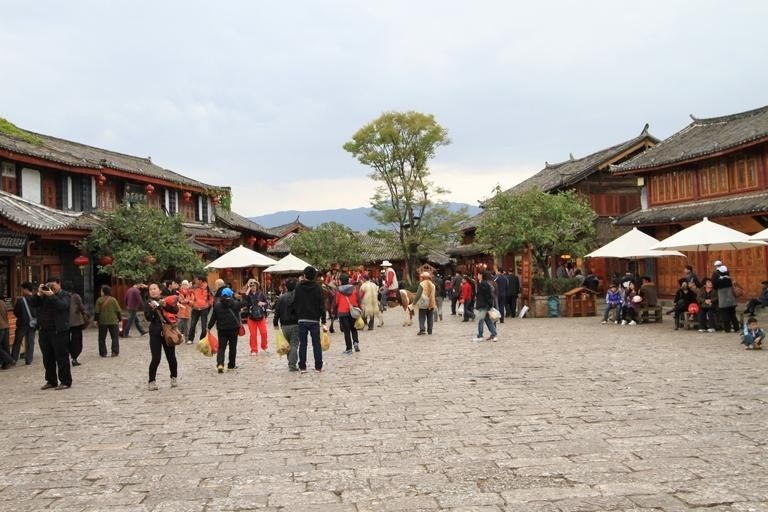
[222,288,233,297]
[420,271,432,279]
[379,260,392,267]
[247,279,260,288]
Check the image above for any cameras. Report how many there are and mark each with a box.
[41,283,51,291]
[148,300,160,309]
[249,281,254,286]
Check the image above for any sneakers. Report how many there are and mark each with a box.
[217,365,223,373]
[171,377,177,387]
[149,381,158,390]
[354,342,359,353]
[56,384,68,389]
[42,384,52,389]
[416,330,426,335]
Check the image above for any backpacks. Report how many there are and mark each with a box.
[249,291,262,320]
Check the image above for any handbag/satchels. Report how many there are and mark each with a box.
[350,306,361,319]
[417,296,429,310]
[29,319,37,327]
[162,324,182,346]
[239,326,245,336]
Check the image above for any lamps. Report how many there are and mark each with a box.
[636,174,645,190]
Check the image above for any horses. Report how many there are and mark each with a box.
[354,281,416,327]
[315,278,338,333]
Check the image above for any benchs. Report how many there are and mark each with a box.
[679,308,744,331]
[627,305,663,322]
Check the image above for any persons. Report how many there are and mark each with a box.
[411,265,521,342]
[241,261,402,374]
[542,262,767,350]
[0,279,245,390]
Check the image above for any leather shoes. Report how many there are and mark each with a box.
[73,359,80,365]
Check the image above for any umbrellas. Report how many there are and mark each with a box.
[204,244,278,300]
[262,251,320,276]
[649,217,767,280]
[747,225,768,243]
[583,227,687,296]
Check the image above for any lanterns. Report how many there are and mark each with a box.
[211,198,219,206]
[182,192,192,204]
[75,256,89,275]
[100,256,113,266]
[95,174,107,186]
[246,234,275,249]
[144,184,154,198]
